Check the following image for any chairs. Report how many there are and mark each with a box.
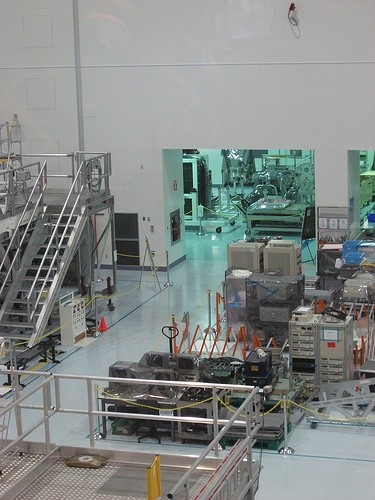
[134,398,172,444]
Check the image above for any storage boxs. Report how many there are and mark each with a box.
[176,382,222,445]
[183,155,209,220]
[222,237,375,392]
[243,349,273,394]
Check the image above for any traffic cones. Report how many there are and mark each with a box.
[98,315,109,332]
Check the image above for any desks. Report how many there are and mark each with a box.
[97,378,190,442]
